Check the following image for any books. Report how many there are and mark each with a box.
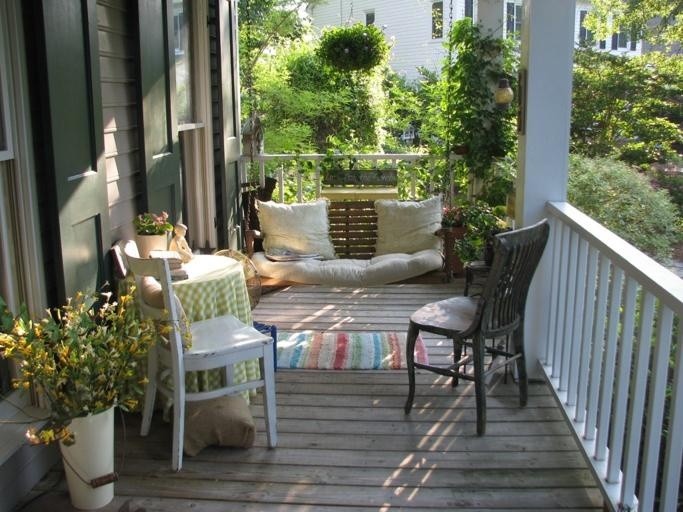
[124,250,189,281]
[264,247,325,261]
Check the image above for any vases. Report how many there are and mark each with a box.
[50,399,118,512]
[137,233,169,260]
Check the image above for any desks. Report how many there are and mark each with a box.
[114,253,262,428]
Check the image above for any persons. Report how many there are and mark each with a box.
[169,225,193,263]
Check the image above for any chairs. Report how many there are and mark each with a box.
[239,196,453,286]
[404,217,550,435]
[122,239,278,472]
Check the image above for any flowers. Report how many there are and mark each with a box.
[133,208,172,234]
[0,284,192,445]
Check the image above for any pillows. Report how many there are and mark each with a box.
[253,200,336,261]
[166,394,255,455]
[376,198,444,255]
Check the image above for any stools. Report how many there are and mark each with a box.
[461,258,497,364]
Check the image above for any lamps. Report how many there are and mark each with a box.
[495,77,514,105]
